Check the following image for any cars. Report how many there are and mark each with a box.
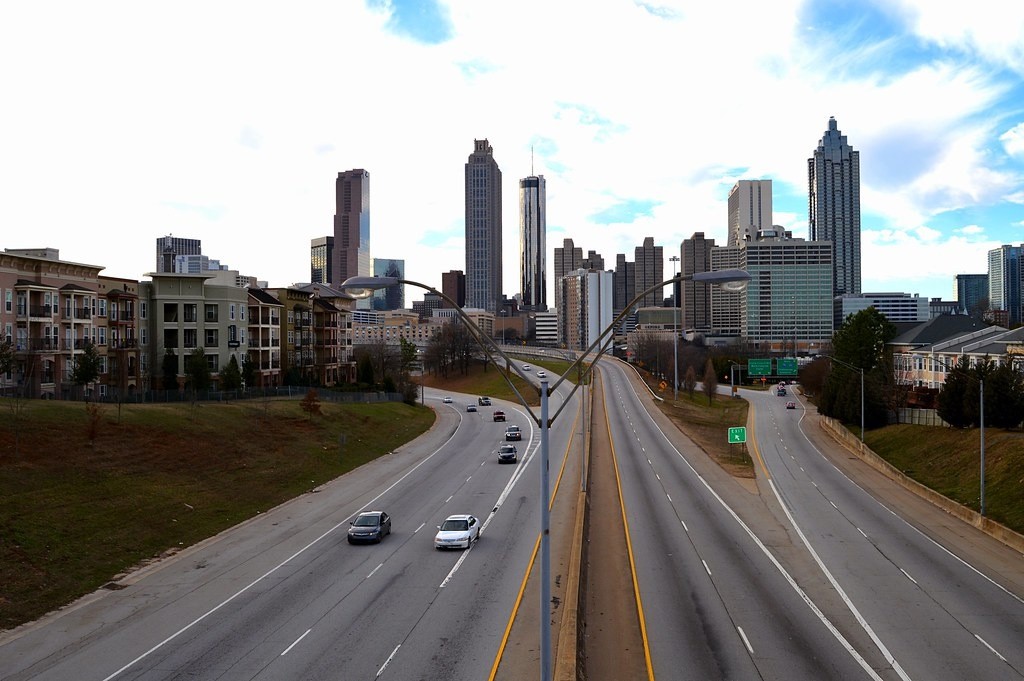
[466,404,477,412]
[346,511,391,545]
[536,371,547,377]
[442,396,453,404]
[776,380,797,397]
[433,514,481,551]
[786,401,796,409]
[521,364,531,371]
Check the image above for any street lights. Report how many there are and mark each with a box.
[728,359,741,387]
[501,308,506,346]
[911,354,986,515]
[581,343,628,493]
[668,256,681,400]
[815,353,864,442]
[341,267,758,681]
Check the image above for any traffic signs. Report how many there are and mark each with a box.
[776,359,799,376]
[748,358,772,376]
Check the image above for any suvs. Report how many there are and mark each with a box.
[493,410,507,421]
[478,395,492,405]
[497,445,518,464]
[505,424,522,442]
